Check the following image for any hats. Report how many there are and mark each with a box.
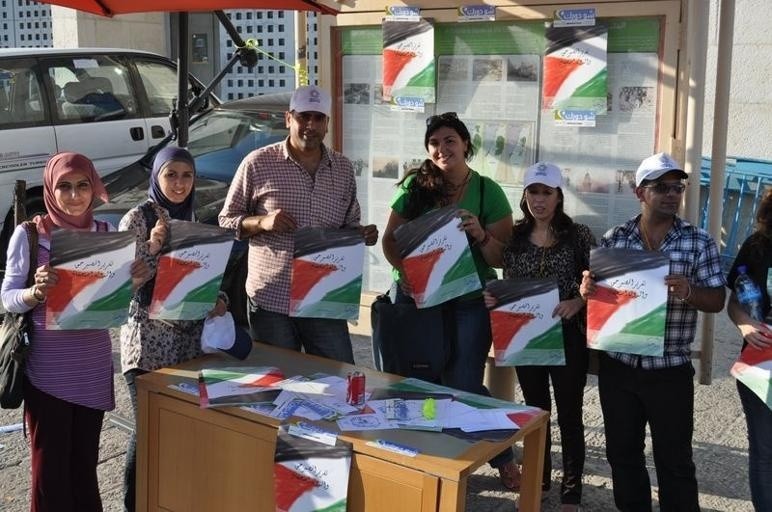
[521,161,564,190]
[201,309,253,361]
[288,83,332,118]
[634,152,688,188]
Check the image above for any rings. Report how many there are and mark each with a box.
[40,278,44,283]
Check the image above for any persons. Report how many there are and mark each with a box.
[483,162,597,505]
[118,147,230,511]
[726,185,771,511]
[0,151,151,512]
[580,153,726,512]
[383,113,524,494]
[217,86,378,364]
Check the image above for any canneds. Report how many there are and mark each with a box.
[345,371,365,405]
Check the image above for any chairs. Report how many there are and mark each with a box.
[25,77,64,117]
[62,82,102,118]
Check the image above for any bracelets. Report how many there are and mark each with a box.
[679,285,693,302]
[480,229,490,247]
[30,284,47,304]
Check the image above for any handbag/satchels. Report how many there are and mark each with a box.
[0,218,39,411]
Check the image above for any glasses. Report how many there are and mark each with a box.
[424,111,458,126]
[646,182,686,194]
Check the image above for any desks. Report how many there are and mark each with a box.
[134,341,550,512]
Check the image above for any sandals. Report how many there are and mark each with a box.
[500,459,522,491]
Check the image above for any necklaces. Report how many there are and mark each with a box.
[437,173,471,205]
[639,220,671,251]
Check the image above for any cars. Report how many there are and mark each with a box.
[90,93,292,232]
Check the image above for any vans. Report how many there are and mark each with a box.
[0,47,223,229]
